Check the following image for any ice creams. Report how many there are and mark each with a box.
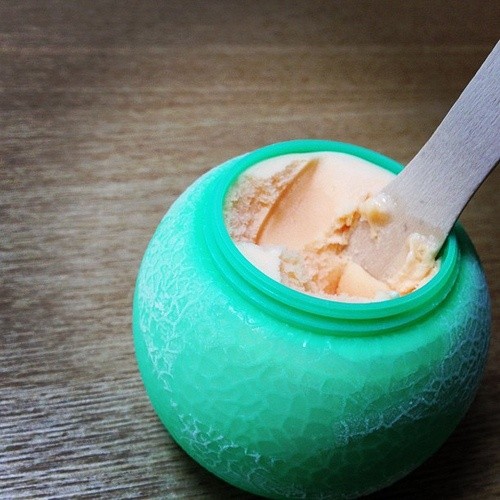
[132,137,490,500]
